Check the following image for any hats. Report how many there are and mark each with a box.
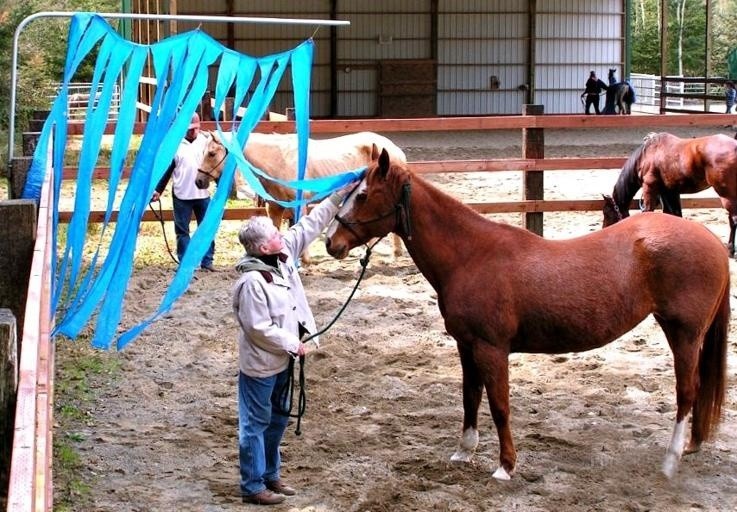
[188,113,200,130]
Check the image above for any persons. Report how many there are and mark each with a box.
[725,81,737,114]
[581,71,610,115]
[233,180,362,505]
[152,113,222,272]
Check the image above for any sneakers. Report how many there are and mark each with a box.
[240,479,296,505]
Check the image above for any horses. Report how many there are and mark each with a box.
[606,69,636,115]
[324,142,733,481]
[600,131,737,262]
[194,128,407,267]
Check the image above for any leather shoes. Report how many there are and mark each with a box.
[200,263,220,272]
